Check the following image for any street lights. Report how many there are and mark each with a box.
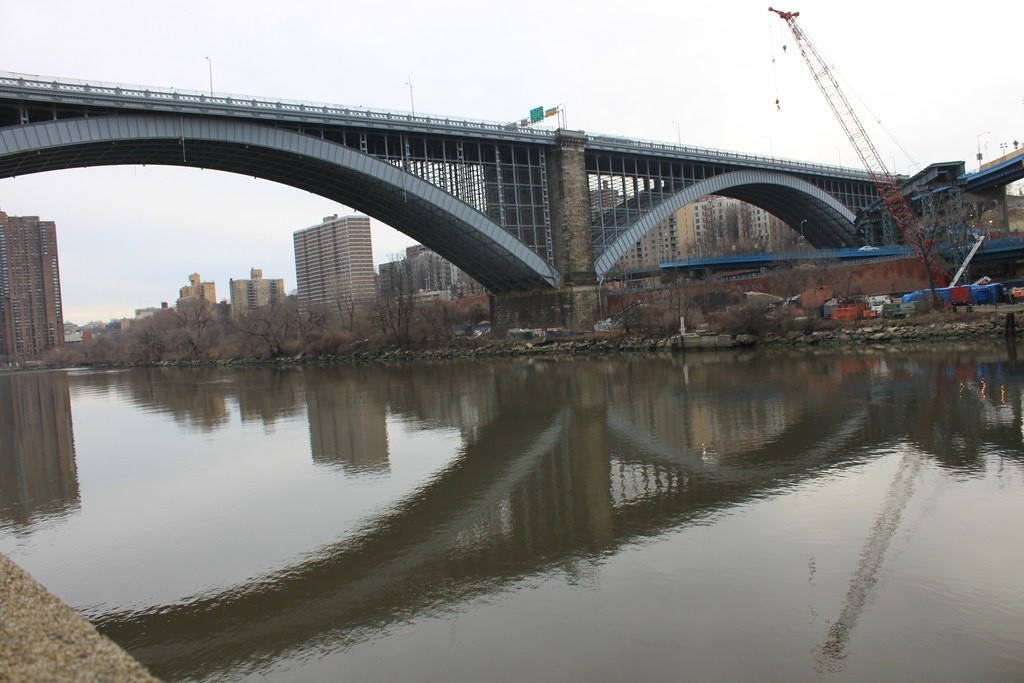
[907,162,921,177]
[405,81,414,119]
[559,103,567,129]
[797,236,804,269]
[766,136,773,163]
[978,131,991,167]
[671,120,682,152]
[888,155,895,173]
[834,148,841,172]
[800,219,807,243]
[206,56,213,102]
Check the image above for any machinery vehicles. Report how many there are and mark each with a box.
[768,6,973,307]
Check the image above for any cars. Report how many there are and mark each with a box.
[858,246,879,253]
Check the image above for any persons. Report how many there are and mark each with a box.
[952,298,958,312]
